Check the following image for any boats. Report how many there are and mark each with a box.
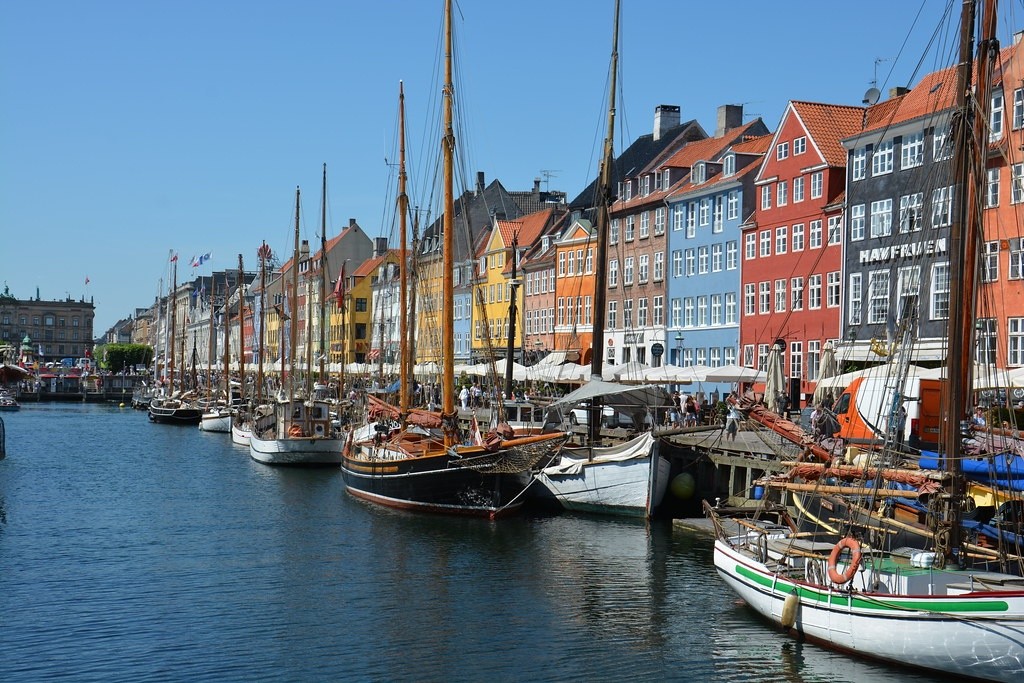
[0,391,20,411]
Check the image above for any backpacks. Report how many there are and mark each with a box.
[694,401,700,412]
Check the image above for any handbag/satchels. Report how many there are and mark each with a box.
[467,392,471,400]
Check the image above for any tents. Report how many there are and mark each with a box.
[370,361,788,383]
[886,399,908,451]
[819,363,1024,389]
[185,359,368,375]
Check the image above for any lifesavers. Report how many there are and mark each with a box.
[828,537,861,584]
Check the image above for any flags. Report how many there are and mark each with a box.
[192,287,198,297]
[334,265,344,313]
[85,275,89,284]
[192,251,212,266]
[86,349,91,357]
[170,251,178,261]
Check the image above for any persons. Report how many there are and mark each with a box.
[331,383,531,410]
[774,390,792,422]
[725,396,739,441]
[971,405,986,426]
[810,394,838,435]
[669,389,717,429]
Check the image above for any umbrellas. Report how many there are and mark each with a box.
[544,381,653,446]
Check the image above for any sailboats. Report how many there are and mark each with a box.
[128,0,1024,683]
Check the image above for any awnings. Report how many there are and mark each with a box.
[188,256,198,276]
